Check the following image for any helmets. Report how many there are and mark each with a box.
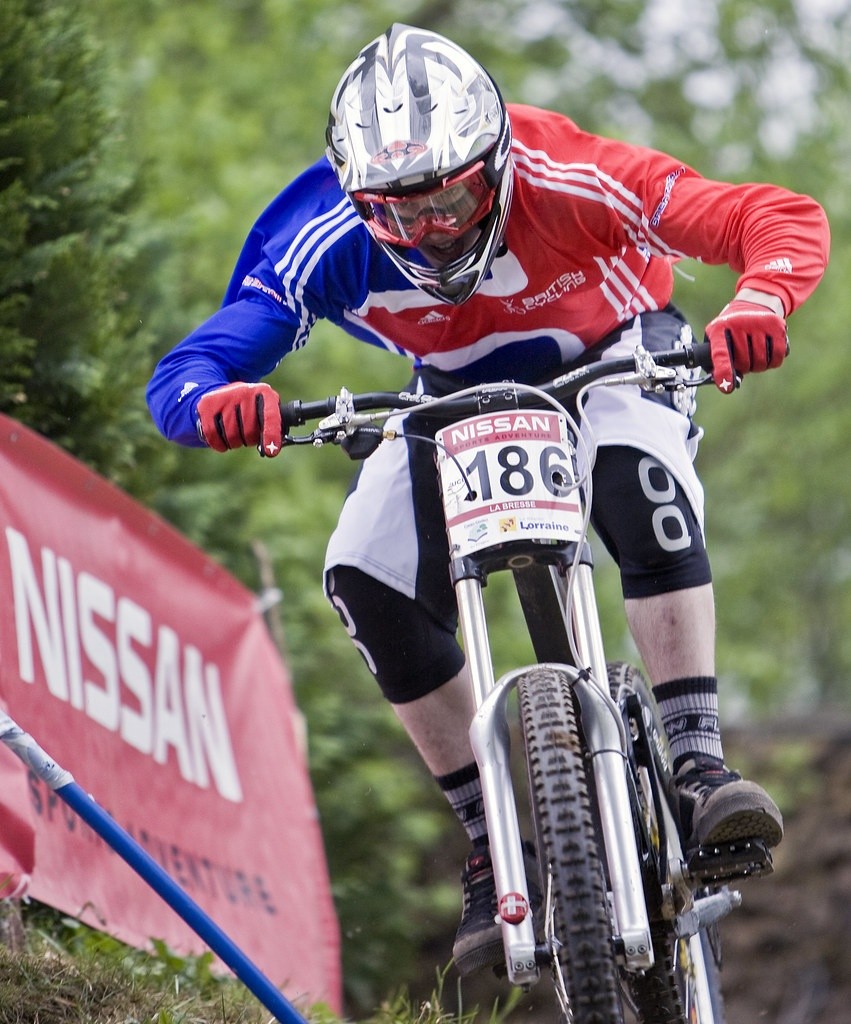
[324,23,514,308]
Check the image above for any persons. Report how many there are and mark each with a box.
[147,21,831,977]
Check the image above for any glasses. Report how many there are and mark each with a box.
[355,63,512,248]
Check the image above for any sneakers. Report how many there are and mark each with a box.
[451,839,542,981]
[674,755,782,852]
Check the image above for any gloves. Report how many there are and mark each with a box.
[196,380,280,457]
[704,300,786,395]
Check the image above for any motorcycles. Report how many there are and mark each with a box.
[197,335,790,1024]
